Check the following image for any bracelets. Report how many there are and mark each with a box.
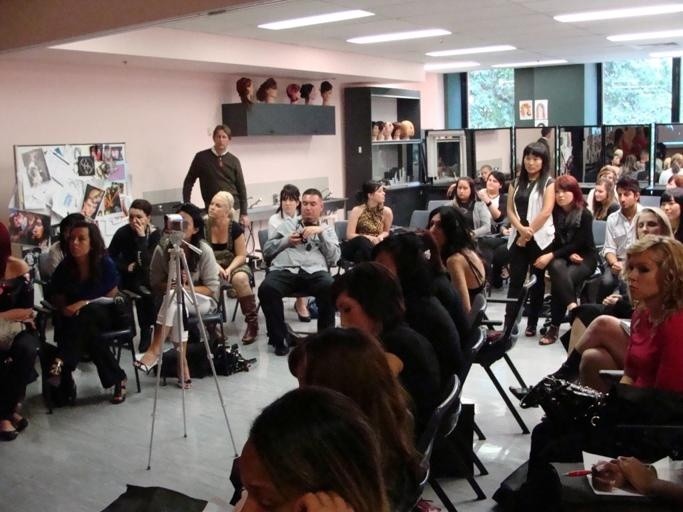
[85,300,89,306]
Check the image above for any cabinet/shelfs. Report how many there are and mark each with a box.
[344,86,425,226]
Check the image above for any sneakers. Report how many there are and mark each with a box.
[539,325,560,345]
[274,333,296,356]
[138,328,152,352]
[524,324,537,338]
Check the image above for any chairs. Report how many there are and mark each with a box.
[407,461,431,512]
[467,292,487,327]
[258,229,268,267]
[140,278,233,386]
[34,304,53,416]
[589,220,606,277]
[473,274,539,434]
[417,374,487,511]
[334,220,349,242]
[427,200,453,211]
[40,289,141,394]
[410,210,430,232]
[454,324,489,475]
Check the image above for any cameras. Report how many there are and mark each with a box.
[163,213,188,234]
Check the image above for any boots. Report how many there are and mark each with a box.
[208,320,227,340]
[237,294,260,343]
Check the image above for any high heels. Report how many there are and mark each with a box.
[176,366,191,389]
[133,355,164,376]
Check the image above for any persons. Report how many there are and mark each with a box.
[393,122,402,140]
[182,123,250,299]
[236,77,254,104]
[372,122,379,140]
[383,121,394,140]
[301,84,316,105]
[378,120,385,140]
[401,120,414,139]
[257,77,278,104]
[320,81,333,105]
[286,84,299,105]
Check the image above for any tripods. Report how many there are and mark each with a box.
[147,248,238,471]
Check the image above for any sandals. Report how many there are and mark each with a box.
[0,426,19,439]
[15,417,29,430]
[111,370,130,403]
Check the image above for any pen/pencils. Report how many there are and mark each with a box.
[562,470,592,477]
